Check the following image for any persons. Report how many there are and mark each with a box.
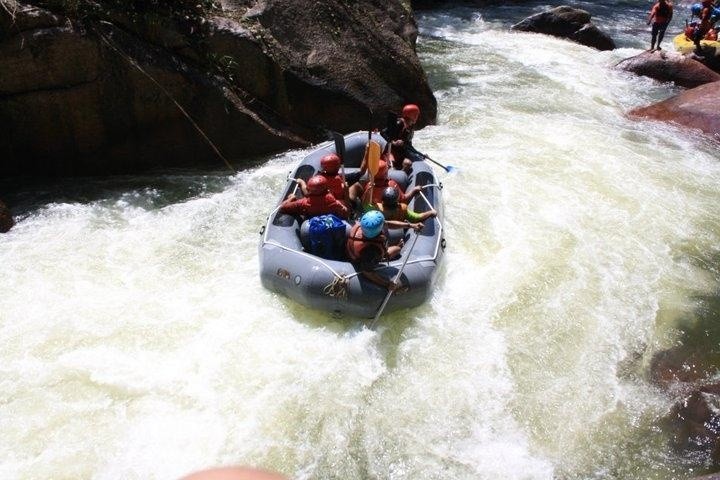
[691,0,720,51]
[647,0,673,50]
[279,104,437,291]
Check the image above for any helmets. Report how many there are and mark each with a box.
[374,159,389,180]
[360,209,386,239]
[306,174,328,196]
[402,104,421,122]
[381,186,401,208]
[319,153,342,176]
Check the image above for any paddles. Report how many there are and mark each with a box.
[333,132,345,185]
[368,141,380,203]
[417,152,452,173]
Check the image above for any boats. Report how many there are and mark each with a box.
[673,32,720,60]
[259,130,446,317]
